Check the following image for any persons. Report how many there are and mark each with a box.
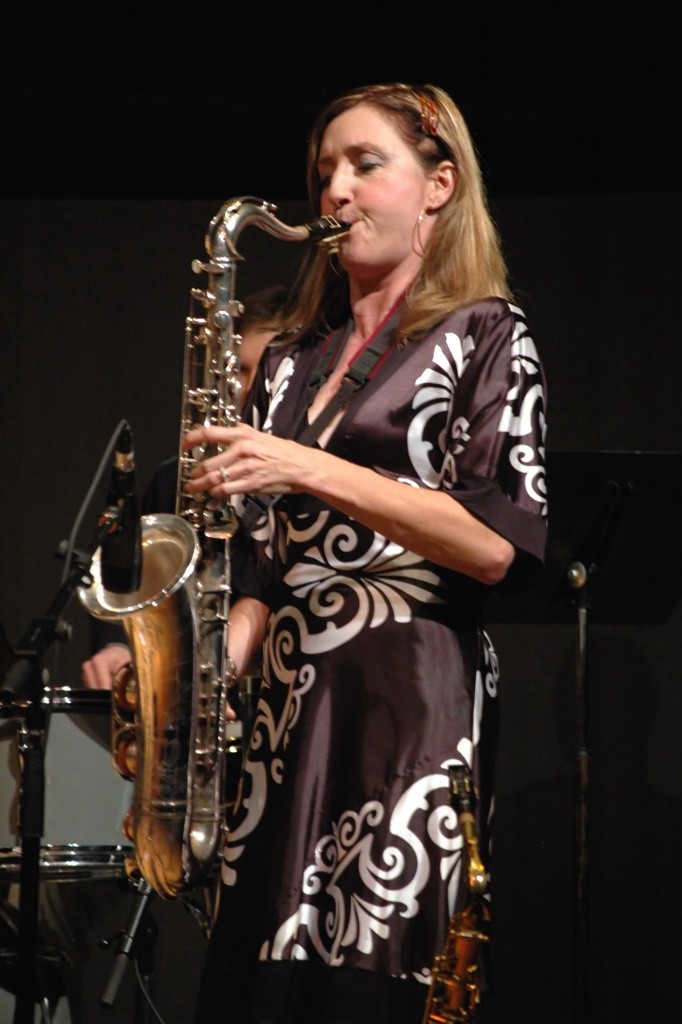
[85,284,308,1024]
[181,84,546,1024]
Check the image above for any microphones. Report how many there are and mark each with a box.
[100,426,143,592]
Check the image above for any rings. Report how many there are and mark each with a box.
[218,466,229,484]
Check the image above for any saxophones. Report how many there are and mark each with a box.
[76,196,354,943]
[420,764,493,1024]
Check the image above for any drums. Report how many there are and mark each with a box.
[0,843,136,1004]
